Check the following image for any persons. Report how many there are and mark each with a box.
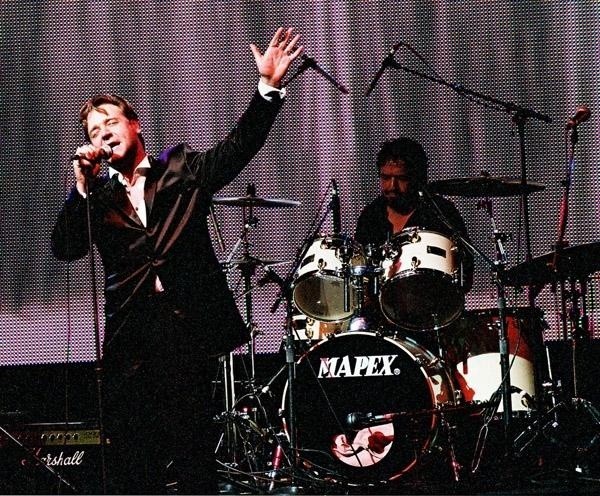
[349,139,474,297]
[49,25,306,493]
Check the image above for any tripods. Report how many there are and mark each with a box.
[259,268,348,496]
[219,209,286,495]
[471,202,600,486]
[211,343,278,495]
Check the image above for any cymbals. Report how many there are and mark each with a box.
[505,241,600,287]
[426,177,547,197]
[214,195,301,208]
[220,255,289,265]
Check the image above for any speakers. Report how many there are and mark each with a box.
[0,422,106,495]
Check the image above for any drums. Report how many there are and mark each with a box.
[380,229,465,333]
[280,330,437,489]
[439,306,542,412]
[293,233,366,323]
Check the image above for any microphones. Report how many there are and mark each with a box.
[416,190,425,230]
[364,41,403,97]
[565,105,591,128]
[302,54,349,95]
[260,261,284,287]
[345,411,399,430]
[268,432,290,492]
[331,178,341,233]
[70,144,114,161]
[342,260,351,313]
